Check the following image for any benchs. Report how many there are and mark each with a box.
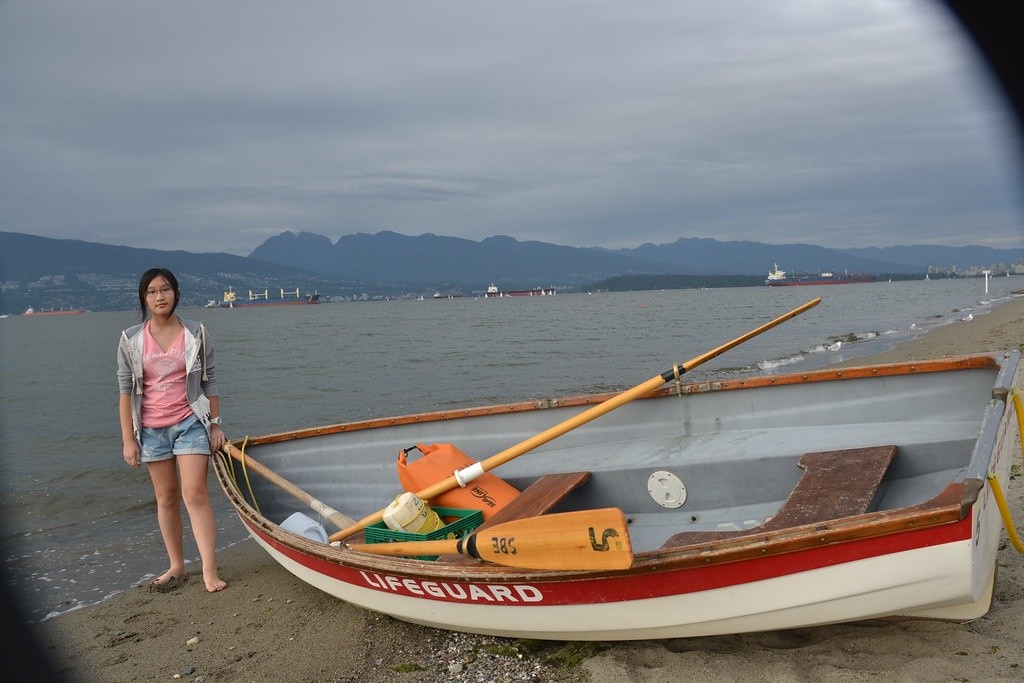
[438,471,592,562]
[661,443,899,551]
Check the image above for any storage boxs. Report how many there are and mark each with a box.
[365,507,485,561]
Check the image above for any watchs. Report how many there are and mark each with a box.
[211,417,222,425]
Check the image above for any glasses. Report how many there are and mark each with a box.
[146,287,173,297]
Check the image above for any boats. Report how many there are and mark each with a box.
[221,289,323,304]
[765,262,875,287]
[23,306,86,316]
[211,350,1020,642]
[486,284,555,297]
[205,300,219,308]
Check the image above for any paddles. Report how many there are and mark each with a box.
[324,291,827,543]
[345,506,636,573]
[221,441,367,546]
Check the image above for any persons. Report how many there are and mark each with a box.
[117,268,229,594]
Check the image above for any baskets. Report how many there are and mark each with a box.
[364,507,484,561]
